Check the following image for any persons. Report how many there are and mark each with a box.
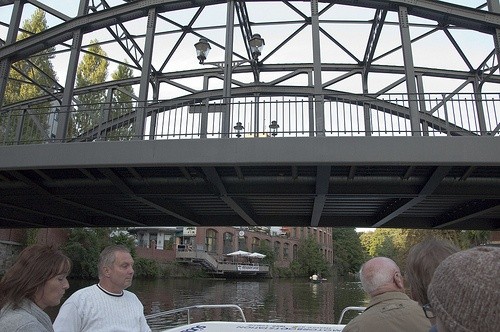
[52,245,152,332]
[405,238,459,306]
[342,257,433,332]
[0,244,71,332]
[423,247,500,332]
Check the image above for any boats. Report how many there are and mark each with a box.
[312,277,328,283]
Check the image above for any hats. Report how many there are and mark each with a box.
[427,248,500,332]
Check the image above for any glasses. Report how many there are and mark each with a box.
[423,304,436,319]
